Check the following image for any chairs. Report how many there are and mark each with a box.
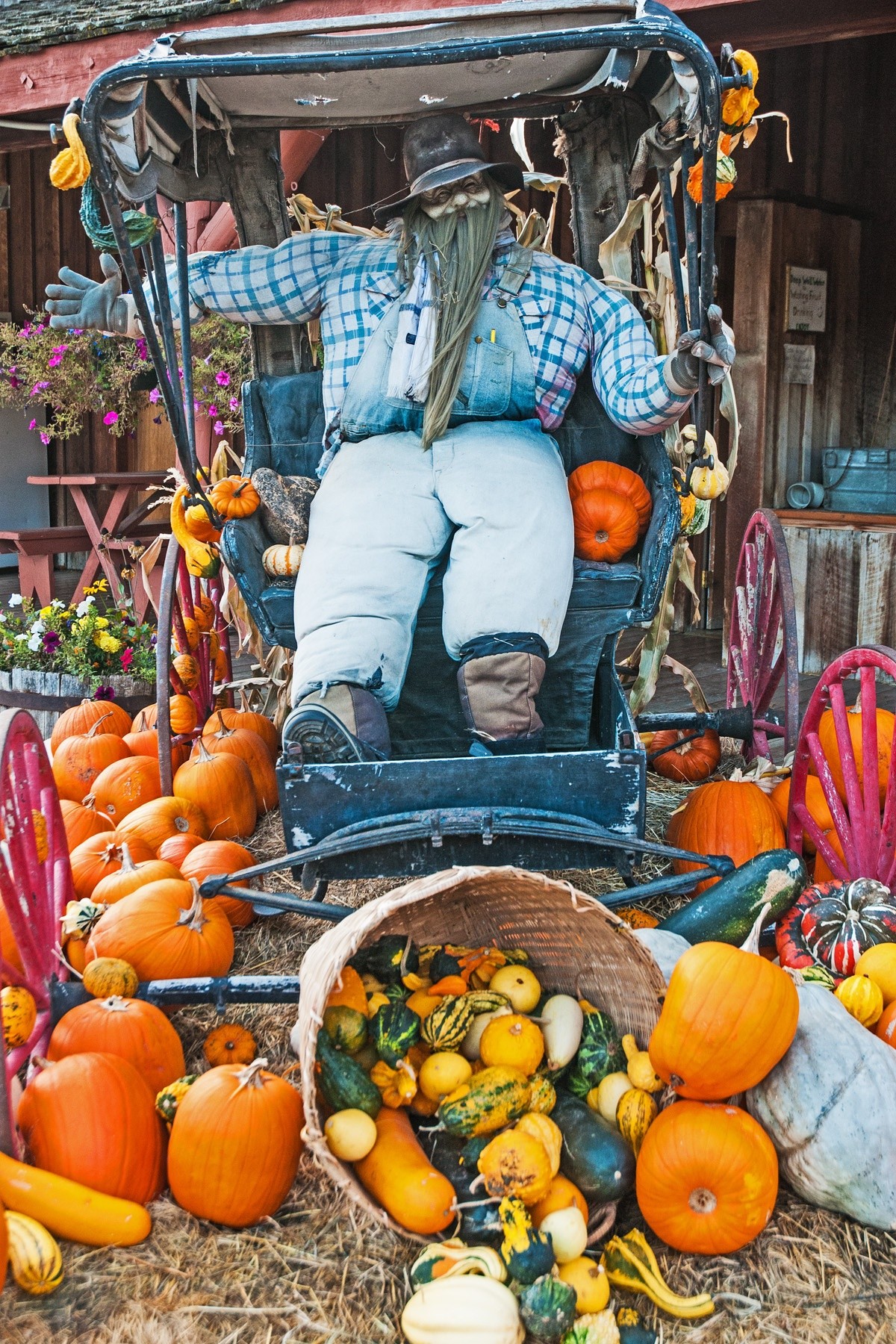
[214,361,682,647]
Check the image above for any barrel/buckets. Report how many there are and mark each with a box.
[822,446,896,516]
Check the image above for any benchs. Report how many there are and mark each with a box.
[105,533,170,627]
[0,518,172,615]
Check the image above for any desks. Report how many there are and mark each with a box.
[27,469,181,622]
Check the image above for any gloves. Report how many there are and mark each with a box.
[670,304,738,392]
[44,251,143,340]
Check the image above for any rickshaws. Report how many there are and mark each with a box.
[47,4,759,1043]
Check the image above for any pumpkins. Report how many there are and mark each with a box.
[1,461,896,1299]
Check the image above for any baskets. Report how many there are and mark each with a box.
[299,865,676,1263]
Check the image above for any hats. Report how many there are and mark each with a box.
[374,113,526,226]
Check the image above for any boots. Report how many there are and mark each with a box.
[457,632,550,758]
[280,680,392,765]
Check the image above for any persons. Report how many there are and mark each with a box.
[41,103,735,767]
